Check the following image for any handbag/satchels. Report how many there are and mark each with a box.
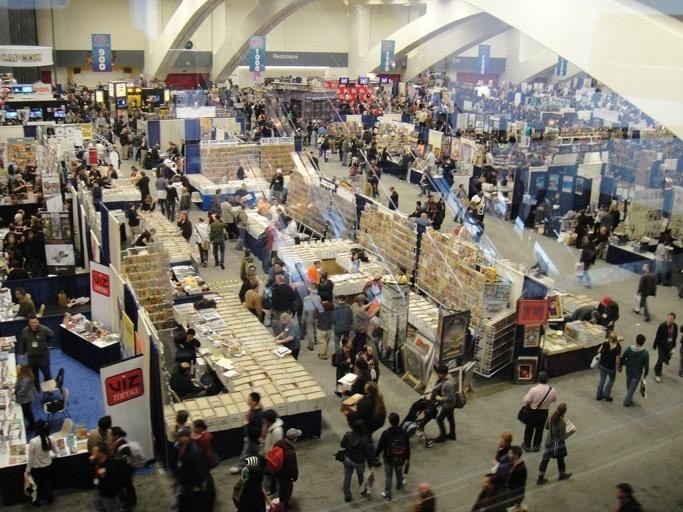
[589,351,602,369]
[455,390,467,409]
[640,377,650,397]
[516,383,554,425]
[543,429,569,458]
[665,349,675,365]
[332,430,354,463]
[193,223,211,252]
[22,469,37,504]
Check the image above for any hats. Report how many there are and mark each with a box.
[286,427,303,440]
[172,428,189,437]
[243,456,258,467]
[276,167,283,175]
[333,295,346,302]
[601,293,615,307]
[372,272,382,281]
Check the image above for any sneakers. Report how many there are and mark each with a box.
[521,441,530,451]
[397,476,407,487]
[434,433,446,442]
[446,430,456,440]
[558,472,572,481]
[305,343,314,351]
[345,492,353,503]
[603,395,614,403]
[228,466,241,474]
[655,372,660,382]
[597,393,601,400]
[537,473,548,484]
[630,308,643,316]
[381,489,389,501]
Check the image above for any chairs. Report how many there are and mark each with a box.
[40,368,64,402]
[34,303,46,317]
[42,388,71,422]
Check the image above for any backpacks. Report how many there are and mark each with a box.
[242,257,255,274]
[266,444,288,478]
[233,210,243,225]
[117,438,147,468]
[93,187,101,199]
[385,430,406,468]
[370,394,387,429]
[8,164,15,176]
[257,227,274,248]
[331,350,340,368]
[232,466,250,509]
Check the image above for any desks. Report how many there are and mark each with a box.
[172,263,210,304]
[605,233,672,284]
[410,167,442,192]
[484,195,512,220]
[384,156,400,175]
[58,319,120,373]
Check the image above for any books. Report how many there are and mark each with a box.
[196,307,246,380]
[274,345,293,359]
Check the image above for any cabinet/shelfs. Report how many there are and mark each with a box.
[473,309,517,379]
[326,192,357,241]
[0,124,25,147]
[0,179,71,226]
[0,286,18,336]
[184,174,270,211]
[199,139,238,183]
[164,383,327,469]
[414,226,512,316]
[27,433,104,491]
[137,210,192,264]
[496,258,555,296]
[36,122,94,144]
[277,239,361,268]
[91,164,142,223]
[229,205,295,262]
[328,122,362,153]
[0,337,27,508]
[541,320,624,378]
[121,248,173,331]
[358,191,417,279]
[238,144,260,167]
[172,293,322,391]
[628,191,667,233]
[282,170,327,240]
[408,290,474,352]
[374,119,416,158]
[260,138,295,179]
[329,251,382,295]
[552,288,601,321]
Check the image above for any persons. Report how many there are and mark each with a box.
[343,357,371,396]
[14,213,27,243]
[55,81,120,188]
[6,260,30,282]
[21,219,37,243]
[294,277,310,341]
[354,343,380,385]
[291,262,306,283]
[176,474,214,512]
[522,370,557,453]
[172,428,200,512]
[269,169,284,198]
[239,265,260,300]
[537,402,577,484]
[261,289,274,326]
[155,173,169,214]
[307,259,322,289]
[234,203,251,251]
[261,221,282,275]
[595,332,621,402]
[168,351,208,402]
[6,245,24,271]
[211,188,222,214]
[272,428,303,512]
[190,419,216,497]
[3,224,21,243]
[258,197,273,216]
[317,270,334,302]
[272,312,301,361]
[80,441,121,512]
[633,264,656,323]
[229,392,264,475]
[237,455,267,512]
[177,210,192,244]
[478,431,514,501]
[301,284,325,352]
[109,426,138,509]
[136,170,150,204]
[87,415,115,457]
[608,199,620,231]
[311,301,334,360]
[375,411,411,502]
[410,73,524,199]
[172,410,192,488]
[600,207,614,232]
[339,419,372,502]
[208,207,223,225]
[61,183,70,204]
[13,286,37,319]
[163,179,179,222]
[542,180,561,218]
[209,214,227,270]
[221,170,232,185]
[367,315,384,359]
[464,200,478,219]
[363,273,383,317]
[10,173,27,194]
[195,76,277,148]
[171,174,184,218]
[288,72,410,210]
[652,312,678,382]
[7,158,18,182]
[270,255,288,272]
[423,194,438,225]
[179,184,191,216]
[120,73,186,183]
[502,445,527,512]
[335,336,355,399]
[574,208,588,249]
[1,232,21,258]
[241,272,259,308]
[471,191,485,230]
[396,264,408,285]
[22,232,47,278]
[597,294,619,332]
[411,482,435,512]
[590,309,613,339]
[432,194,446,230]
[170,279,187,298]
[350,251,361,273]
[268,199,286,222]
[233,182,248,206]
[330,295,353,352]
[125,202,141,247]
[17,166,27,181]
[174,328,201,365]
[351,293,375,354]
[270,274,296,320]
[615,483,644,512]
[678,324,683,378]
[273,207,287,231]
[569,304,597,322]
[25,419,59,508]
[343,381,384,469]
[282,215,300,245]
[409,200,426,218]
[264,261,290,290]
[240,249,257,283]
[619,334,650,407]
[463,206,484,243]
[524,80,683,189]
[593,204,608,237]
[20,229,35,279]
[142,194,157,211]
[12,360,37,433]
[132,227,157,248]
[664,228,678,247]
[577,235,598,290]
[14,208,25,223]
[193,216,211,269]
[245,280,262,322]
[592,225,608,251]
[220,195,235,240]
[426,362,457,444]
[258,408,284,497]
[453,184,468,225]
[655,235,674,286]
[91,181,103,212]
[470,472,507,512]
[19,314,54,394]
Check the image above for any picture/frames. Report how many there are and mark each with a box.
[514,358,537,384]
[438,309,471,362]
[448,366,463,394]
[399,333,436,389]
